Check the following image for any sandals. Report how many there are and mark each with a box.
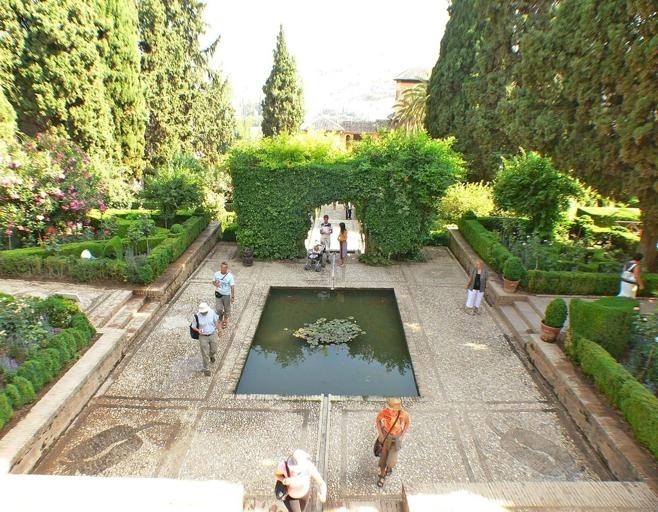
[387,468,392,475]
[377,476,385,487]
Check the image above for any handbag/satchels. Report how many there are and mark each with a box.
[275,480,288,500]
[190,324,198,339]
[215,291,221,298]
[621,271,636,282]
[374,439,382,456]
[465,275,473,288]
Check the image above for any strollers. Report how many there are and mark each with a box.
[305,239,334,272]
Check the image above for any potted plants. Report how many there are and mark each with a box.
[501,256,528,293]
[540,297,568,343]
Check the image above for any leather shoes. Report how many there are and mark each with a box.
[219,320,228,328]
[210,357,216,363]
[204,368,210,377]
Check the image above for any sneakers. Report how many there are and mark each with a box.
[474,310,481,315]
[338,262,346,267]
[466,308,471,313]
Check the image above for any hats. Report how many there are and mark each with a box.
[288,449,309,467]
[198,302,208,313]
[388,398,402,410]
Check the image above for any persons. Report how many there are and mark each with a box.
[376,399,409,487]
[337,222,348,267]
[319,215,331,250]
[213,262,235,327]
[344,203,352,219]
[273,449,327,511]
[464,260,489,315]
[191,303,222,373]
[618,252,645,298]
